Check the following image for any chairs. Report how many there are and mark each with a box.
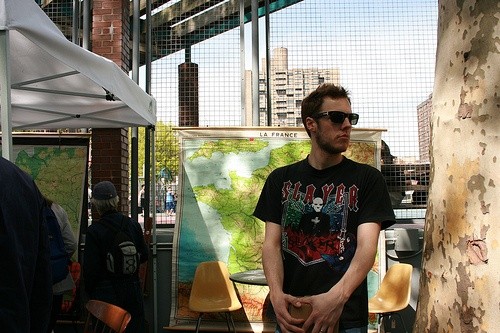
[367,261,414,333]
[82,298,132,333]
[187,259,243,333]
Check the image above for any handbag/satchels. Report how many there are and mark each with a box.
[53,273,75,295]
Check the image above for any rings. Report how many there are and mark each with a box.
[321,329,326,332]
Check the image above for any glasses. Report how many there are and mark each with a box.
[311,111,359,125]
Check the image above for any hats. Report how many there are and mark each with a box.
[93,181,117,200]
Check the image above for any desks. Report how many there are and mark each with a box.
[229,269,269,287]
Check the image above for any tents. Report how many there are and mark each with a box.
[0,1,156,332]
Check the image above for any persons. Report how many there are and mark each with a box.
[83,181,149,332]
[46,197,77,332]
[252,84,396,333]
[166,185,176,217]
[141,184,145,217]
[1,156,76,332]
